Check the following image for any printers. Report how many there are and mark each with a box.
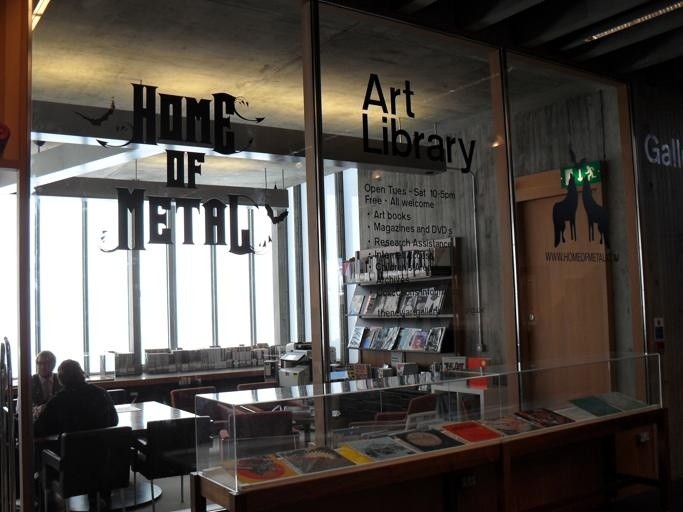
[279,342,336,386]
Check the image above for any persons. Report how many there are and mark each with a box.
[33,359,118,481]
[32,350,62,406]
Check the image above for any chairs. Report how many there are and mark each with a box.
[375,395,437,420]
[35,379,309,512]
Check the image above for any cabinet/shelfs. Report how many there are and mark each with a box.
[431,382,507,420]
[384,387,431,411]
[345,238,464,356]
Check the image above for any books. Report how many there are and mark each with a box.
[115,343,282,375]
[343,250,503,392]
